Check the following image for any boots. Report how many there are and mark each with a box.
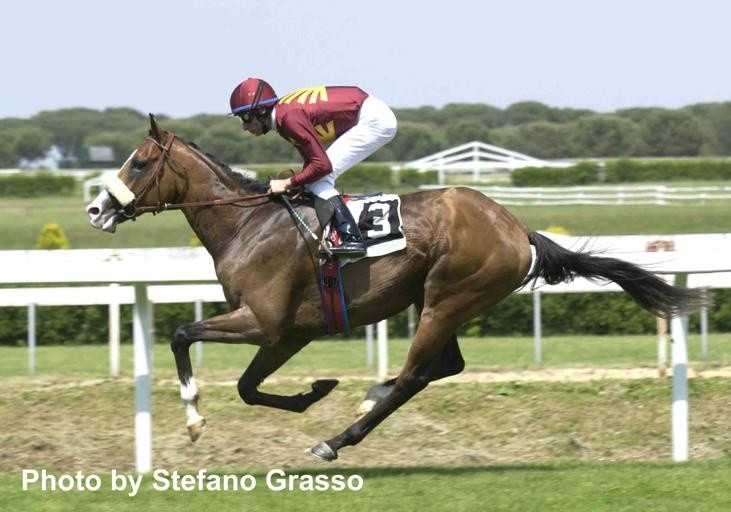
[317,195,367,258]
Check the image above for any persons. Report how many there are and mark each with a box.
[229,77,398,258]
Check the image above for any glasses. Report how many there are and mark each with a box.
[241,111,254,123]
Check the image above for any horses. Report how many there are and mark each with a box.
[84,112,716,463]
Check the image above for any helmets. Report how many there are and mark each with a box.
[230,77,279,114]
